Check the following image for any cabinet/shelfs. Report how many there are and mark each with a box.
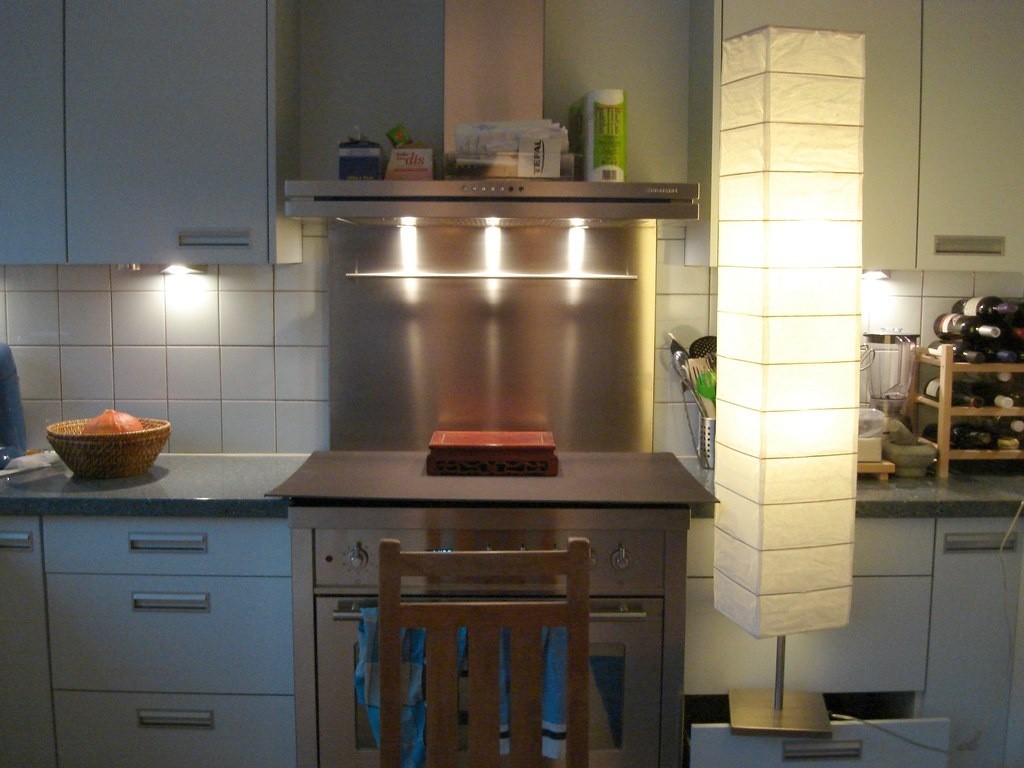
[688,518,1024,768]
[0,0,306,266]
[685,0,1024,276]
[0,516,298,768]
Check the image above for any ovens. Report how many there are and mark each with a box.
[315,599,661,768]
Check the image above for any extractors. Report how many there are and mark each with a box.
[282,180,700,225]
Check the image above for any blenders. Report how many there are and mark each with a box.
[864,327,920,439]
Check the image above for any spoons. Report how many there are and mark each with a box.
[672,352,708,417]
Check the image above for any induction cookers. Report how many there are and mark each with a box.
[263,449,720,595]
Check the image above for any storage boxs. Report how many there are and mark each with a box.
[337,141,382,180]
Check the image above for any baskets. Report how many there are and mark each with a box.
[45,417,172,480]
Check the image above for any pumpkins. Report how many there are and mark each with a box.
[84,410,143,433]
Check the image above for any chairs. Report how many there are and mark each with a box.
[354,537,598,768]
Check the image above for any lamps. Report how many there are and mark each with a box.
[711,24,873,739]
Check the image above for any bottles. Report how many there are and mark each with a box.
[928,295,1024,363]
[924,416,1024,450]
[925,373,1024,407]
